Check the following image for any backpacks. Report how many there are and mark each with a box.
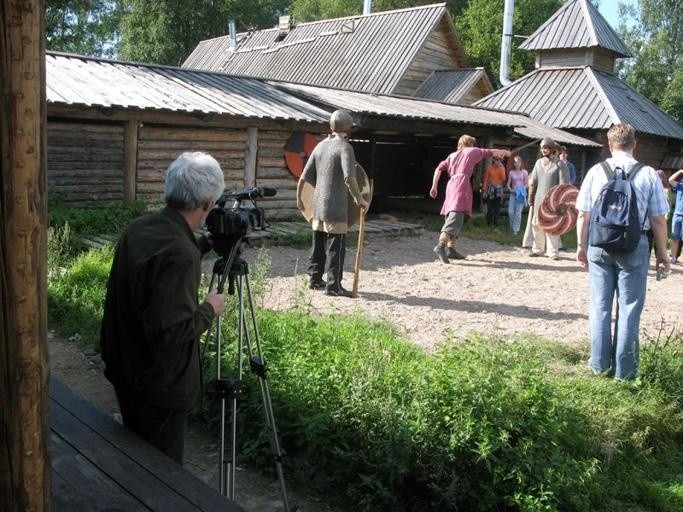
[588,160,649,252]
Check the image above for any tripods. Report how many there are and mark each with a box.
[205,259,291,511]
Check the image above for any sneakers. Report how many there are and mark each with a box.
[310,280,326,290]
[529,252,539,257]
[433,241,450,264]
[326,288,352,297]
[447,247,465,259]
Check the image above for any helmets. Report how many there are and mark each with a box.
[329,109,361,132]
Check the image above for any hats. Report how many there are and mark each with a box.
[540,137,554,148]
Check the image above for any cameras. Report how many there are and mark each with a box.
[189,187,277,260]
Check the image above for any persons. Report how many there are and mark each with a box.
[558,145,577,250]
[99,147,227,470]
[667,169,683,265]
[295,107,372,298]
[644,169,671,268]
[521,141,561,248]
[573,124,672,382]
[505,155,529,236]
[480,146,508,230]
[527,137,568,262]
[428,134,512,267]
